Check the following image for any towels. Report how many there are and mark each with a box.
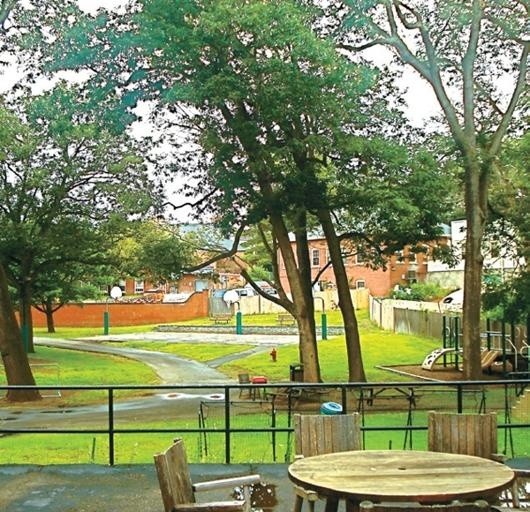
[289,362,304,383]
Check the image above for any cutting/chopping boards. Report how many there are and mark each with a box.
[270,348,277,361]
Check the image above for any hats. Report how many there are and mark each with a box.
[214,279,276,297]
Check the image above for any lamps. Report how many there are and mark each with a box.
[238,372,268,401]
[153,439,260,512]
[293,411,500,512]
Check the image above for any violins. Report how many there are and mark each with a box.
[458,348,498,372]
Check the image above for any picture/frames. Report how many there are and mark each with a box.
[287,450,515,512]
